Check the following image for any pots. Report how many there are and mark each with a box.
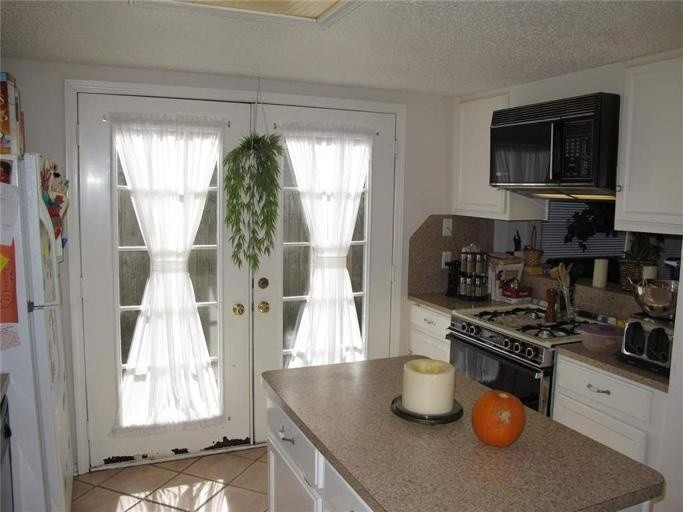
[627,274,679,322]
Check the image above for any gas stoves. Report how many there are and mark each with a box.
[452,303,611,366]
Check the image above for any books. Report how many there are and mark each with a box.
[459,245,524,301]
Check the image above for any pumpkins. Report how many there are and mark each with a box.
[472,390,526,447]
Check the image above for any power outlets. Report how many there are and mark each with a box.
[441,219,453,269]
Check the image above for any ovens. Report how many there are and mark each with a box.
[447,334,555,414]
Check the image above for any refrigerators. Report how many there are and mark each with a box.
[0,148,79,512]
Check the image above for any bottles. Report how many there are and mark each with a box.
[442,246,489,300]
[511,229,523,259]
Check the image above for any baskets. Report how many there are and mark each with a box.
[617,261,659,292]
[523,223,544,266]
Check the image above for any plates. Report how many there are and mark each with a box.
[390,396,464,424]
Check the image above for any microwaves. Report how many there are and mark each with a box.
[486,91,617,199]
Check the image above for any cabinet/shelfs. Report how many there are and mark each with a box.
[265,396,374,510]
[408,302,451,364]
[613,56,683,240]
[548,352,668,479]
[450,92,546,222]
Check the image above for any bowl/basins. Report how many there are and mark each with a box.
[574,323,624,356]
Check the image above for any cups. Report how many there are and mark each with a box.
[590,258,609,289]
[398,359,457,417]
[558,285,576,322]
[641,264,659,280]
